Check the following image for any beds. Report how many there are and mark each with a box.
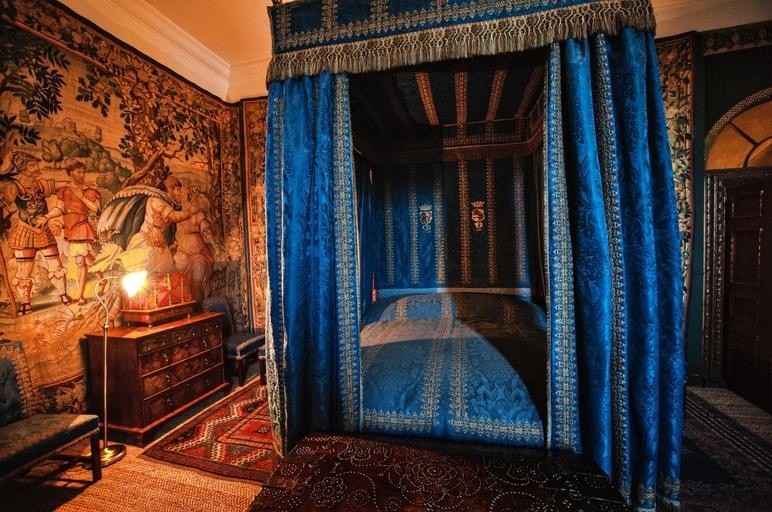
[359,286,548,451]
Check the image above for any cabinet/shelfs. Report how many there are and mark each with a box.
[83,312,230,449]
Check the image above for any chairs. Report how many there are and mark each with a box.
[0,338,102,511]
[201,294,266,387]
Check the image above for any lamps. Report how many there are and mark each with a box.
[93,270,148,468]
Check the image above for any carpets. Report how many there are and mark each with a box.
[135,374,772,512]
